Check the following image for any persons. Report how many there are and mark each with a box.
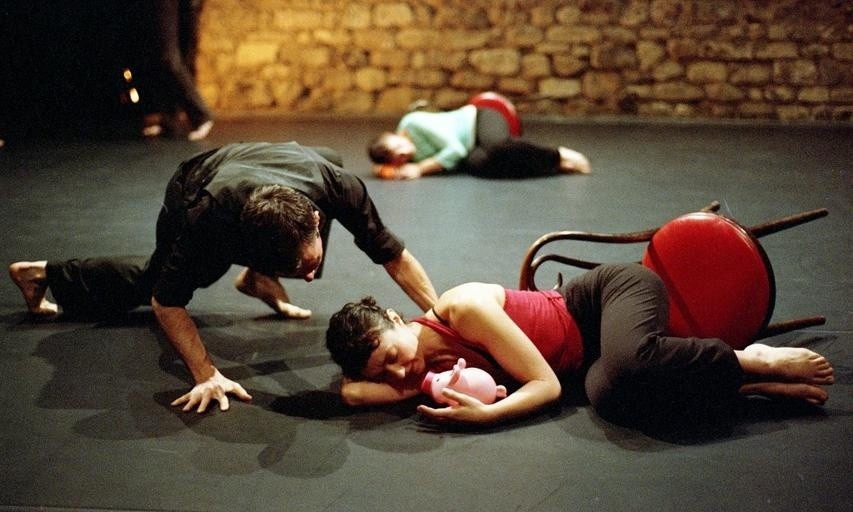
[137,1,217,142]
[368,90,591,180]
[325,262,836,428]
[8,140,439,415]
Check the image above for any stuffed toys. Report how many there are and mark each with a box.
[421,356,508,408]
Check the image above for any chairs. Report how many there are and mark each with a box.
[403,88,553,146]
[517,198,829,348]
